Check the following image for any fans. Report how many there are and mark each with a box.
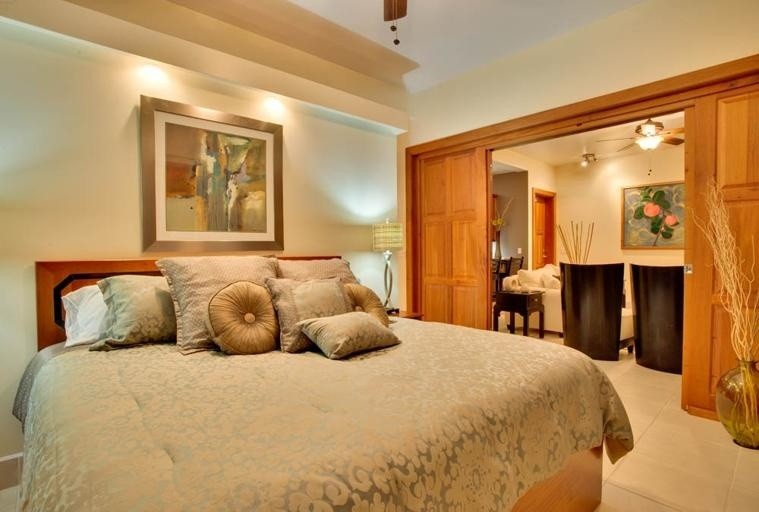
[598,118,685,153]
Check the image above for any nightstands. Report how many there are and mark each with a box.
[385,307,423,321]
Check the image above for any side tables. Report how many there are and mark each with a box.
[491,290,546,339]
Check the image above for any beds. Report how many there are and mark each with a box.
[10,255,635,512]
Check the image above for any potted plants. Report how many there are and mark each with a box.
[682,177,759,449]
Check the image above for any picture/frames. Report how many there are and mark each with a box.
[139,92,286,252]
[621,180,686,250]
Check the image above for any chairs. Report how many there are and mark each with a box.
[492,258,509,290]
[499,256,524,290]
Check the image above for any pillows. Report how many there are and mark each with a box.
[296,309,400,359]
[344,282,389,328]
[61,284,109,347]
[159,256,278,355]
[204,280,278,354]
[90,273,177,351]
[272,257,358,285]
[542,270,562,289]
[265,275,352,353]
[518,268,543,289]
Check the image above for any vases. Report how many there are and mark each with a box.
[495,230,502,260]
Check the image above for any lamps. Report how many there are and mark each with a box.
[384,0,409,49]
[638,123,663,178]
[373,218,405,317]
[579,149,599,169]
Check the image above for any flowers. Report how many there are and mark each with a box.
[492,196,515,232]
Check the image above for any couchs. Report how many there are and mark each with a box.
[503,264,563,338]
